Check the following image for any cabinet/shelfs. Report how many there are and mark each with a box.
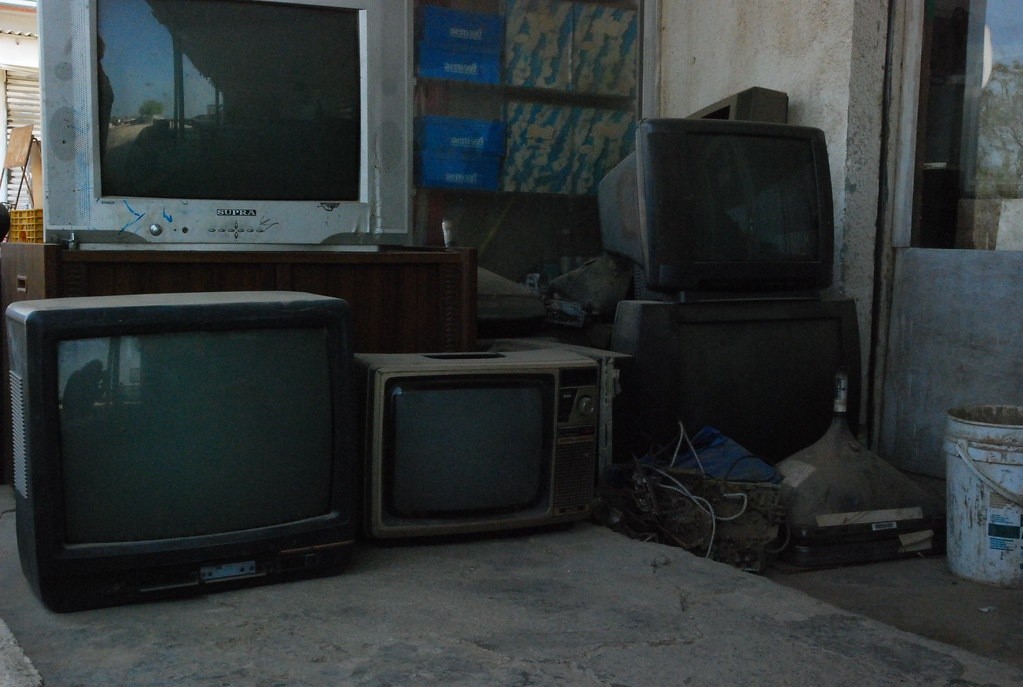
[0,241,479,487]
[413,0,637,215]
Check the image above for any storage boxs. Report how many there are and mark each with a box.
[11,210,44,244]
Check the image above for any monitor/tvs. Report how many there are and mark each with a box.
[6,0,862,610]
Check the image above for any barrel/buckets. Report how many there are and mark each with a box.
[942,404,1023,586]
